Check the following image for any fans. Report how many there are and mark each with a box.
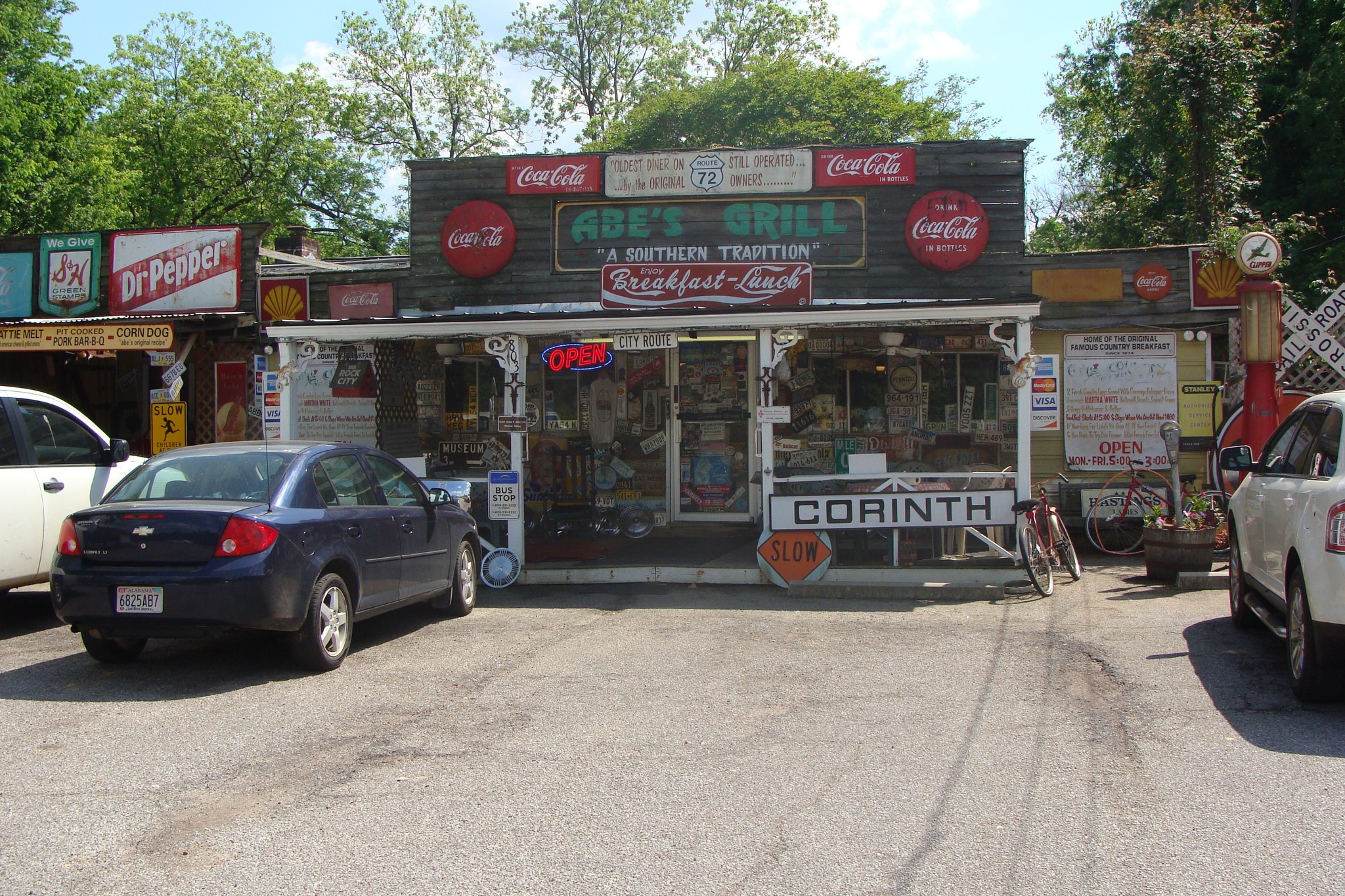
[419,340,485,363]
[860,332,931,358]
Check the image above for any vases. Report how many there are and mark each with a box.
[1143,526,1214,579]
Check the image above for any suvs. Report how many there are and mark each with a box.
[0,386,191,590]
[1219,390,1345,706]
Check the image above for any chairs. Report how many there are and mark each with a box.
[164,481,200,500]
[775,460,1017,557]
[216,479,250,500]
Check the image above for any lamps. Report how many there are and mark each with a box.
[442,357,452,365]
[886,348,897,356]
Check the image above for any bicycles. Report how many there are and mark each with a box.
[1086,460,1232,556]
[1010,472,1081,598]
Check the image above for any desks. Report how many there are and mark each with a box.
[844,482,951,565]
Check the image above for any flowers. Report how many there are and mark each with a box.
[1142,490,1218,532]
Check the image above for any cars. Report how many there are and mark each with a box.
[49,440,483,672]
